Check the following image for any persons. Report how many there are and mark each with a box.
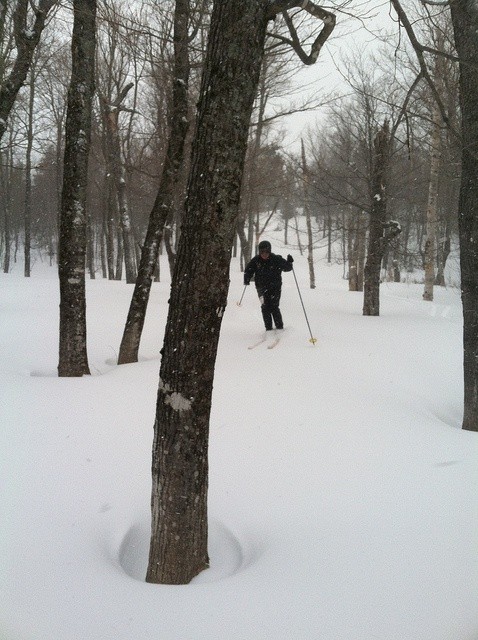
[244,241,293,330]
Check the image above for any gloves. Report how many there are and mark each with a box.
[287,254,293,262]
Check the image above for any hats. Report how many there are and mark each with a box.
[260,241,271,249]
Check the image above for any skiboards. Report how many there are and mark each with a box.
[248,327,288,350]
